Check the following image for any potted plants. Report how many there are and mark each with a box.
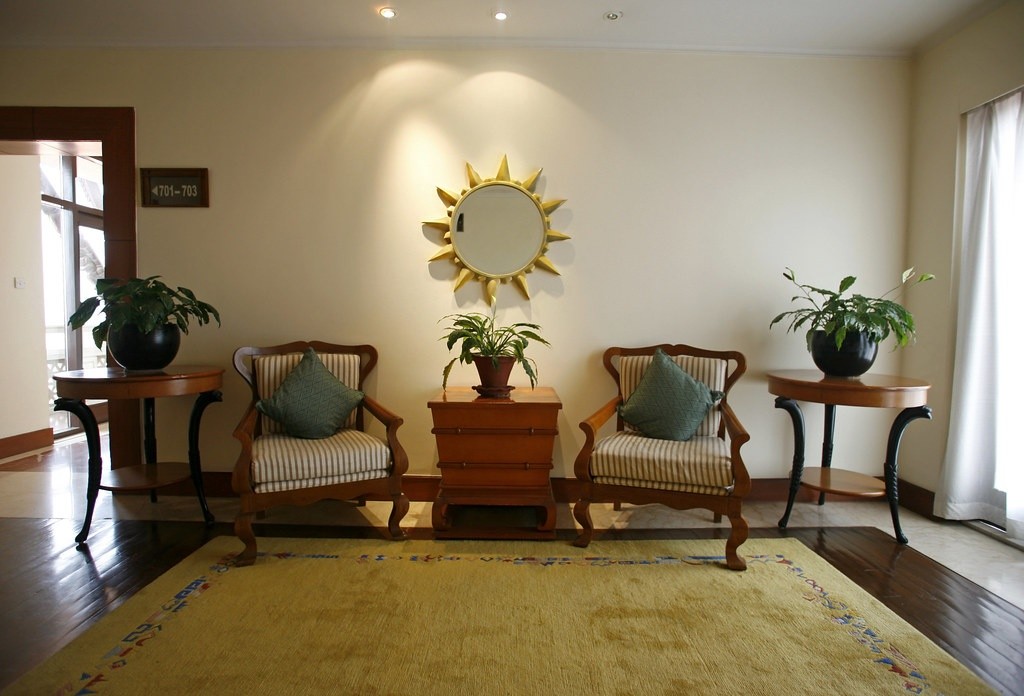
[434,305,551,396]
[67,274,222,370]
[770,267,937,378]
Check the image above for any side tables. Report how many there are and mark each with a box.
[427,387,563,532]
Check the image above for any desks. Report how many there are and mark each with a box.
[768,369,933,543]
[52,365,226,545]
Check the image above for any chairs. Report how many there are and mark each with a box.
[572,344,751,572]
[233,341,410,568]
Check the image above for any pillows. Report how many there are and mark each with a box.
[616,348,723,441]
[255,346,366,440]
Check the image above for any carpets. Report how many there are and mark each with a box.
[0,536,1004,695]
